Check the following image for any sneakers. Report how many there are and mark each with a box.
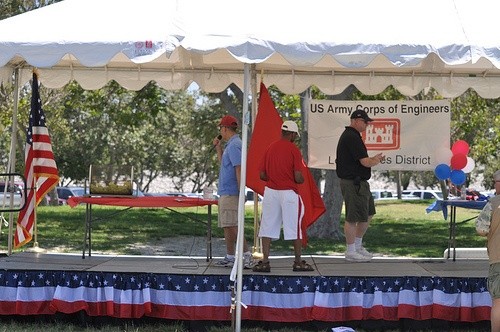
[213,255,235,267]
[345,251,371,262]
[243,253,253,269]
[357,247,373,257]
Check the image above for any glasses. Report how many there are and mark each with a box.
[219,125,225,130]
[492,180,500,186]
[358,120,367,124]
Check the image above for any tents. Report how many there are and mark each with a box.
[0,0,500,332]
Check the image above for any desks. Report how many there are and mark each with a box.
[426,199,489,262]
[67,195,219,262]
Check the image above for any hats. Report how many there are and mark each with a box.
[218,115,238,128]
[281,120,300,138]
[351,110,373,122]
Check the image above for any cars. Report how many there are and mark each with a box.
[369,189,496,201]
[133,189,220,201]
[0,181,25,212]
[55,187,86,204]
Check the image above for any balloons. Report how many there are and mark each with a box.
[435,139,475,185]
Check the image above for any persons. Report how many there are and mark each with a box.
[476,170,500,332]
[252,118,316,272]
[335,109,385,262]
[213,115,255,269]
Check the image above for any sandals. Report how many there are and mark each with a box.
[252,259,271,272]
[293,259,314,271]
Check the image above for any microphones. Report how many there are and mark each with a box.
[209,135,222,154]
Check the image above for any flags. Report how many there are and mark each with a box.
[245,82,327,250]
[14,72,59,249]
